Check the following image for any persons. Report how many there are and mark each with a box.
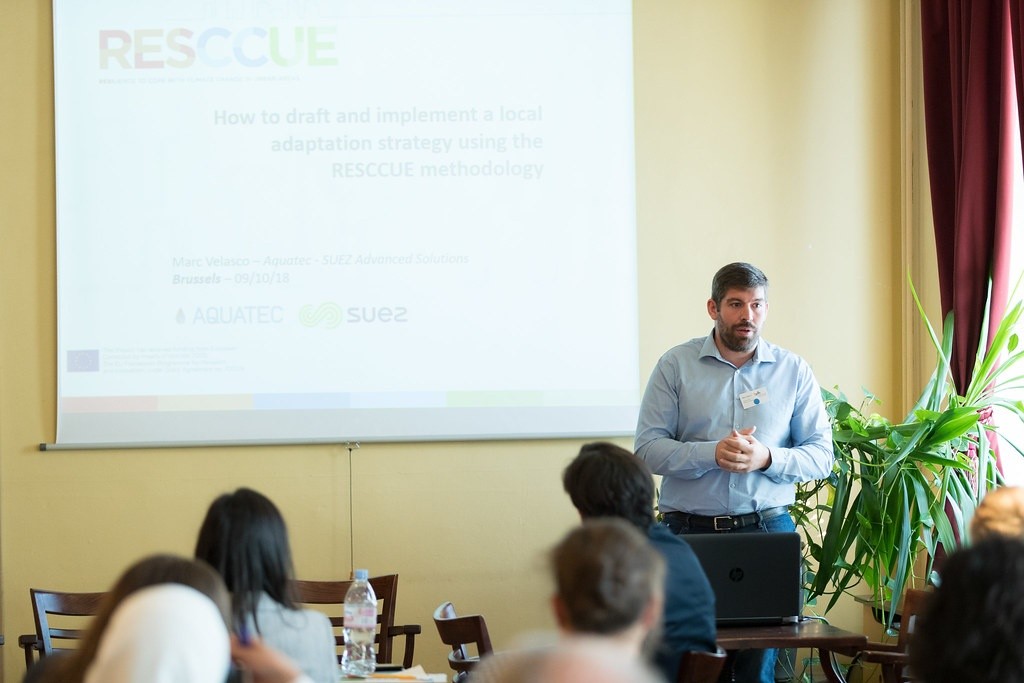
[21,487,341,683]
[562,443,718,683]
[910,487,1024,683]
[633,263,835,683]
[463,518,667,683]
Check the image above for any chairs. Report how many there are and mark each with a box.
[673,645,727,683]
[17,588,110,675]
[284,573,422,676]
[818,589,938,683]
[432,601,495,683]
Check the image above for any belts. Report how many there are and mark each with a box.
[666,506,788,531]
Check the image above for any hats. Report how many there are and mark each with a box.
[82,582,233,683]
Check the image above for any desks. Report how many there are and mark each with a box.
[714,619,867,652]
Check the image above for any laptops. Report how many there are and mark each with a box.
[673,531,808,626]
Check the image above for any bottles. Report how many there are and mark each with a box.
[341,567,377,675]
